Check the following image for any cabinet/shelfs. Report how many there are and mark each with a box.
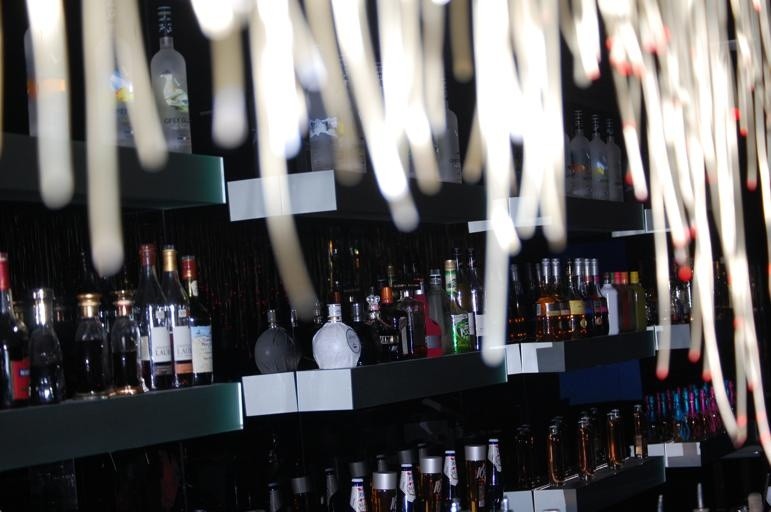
[616,229,736,467]
[508,198,669,512]
[0,135,245,473]
[228,171,512,423]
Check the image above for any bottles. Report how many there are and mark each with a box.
[265,435,514,511]
[564,107,626,205]
[505,252,694,343]
[253,232,486,375]
[24,23,69,140]
[294,70,464,188]
[148,6,194,155]
[2,239,216,403]
[514,380,771,512]
[347,226,507,255]
[93,22,138,146]
